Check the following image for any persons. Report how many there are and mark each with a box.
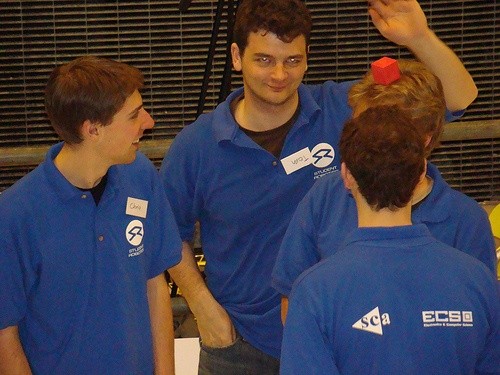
[269,57,500,375]
[0,53,184,375]
[159,0,477,375]
